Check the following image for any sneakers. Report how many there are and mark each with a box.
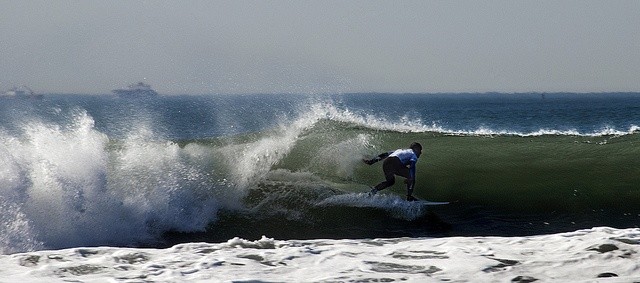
[370,186,376,196]
[407,195,419,203]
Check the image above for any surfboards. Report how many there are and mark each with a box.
[339,182,449,204]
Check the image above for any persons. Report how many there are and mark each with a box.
[362,142,422,202]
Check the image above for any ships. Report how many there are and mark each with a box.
[0,84,46,100]
[108,81,160,99]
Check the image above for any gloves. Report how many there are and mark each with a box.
[362,158,371,165]
